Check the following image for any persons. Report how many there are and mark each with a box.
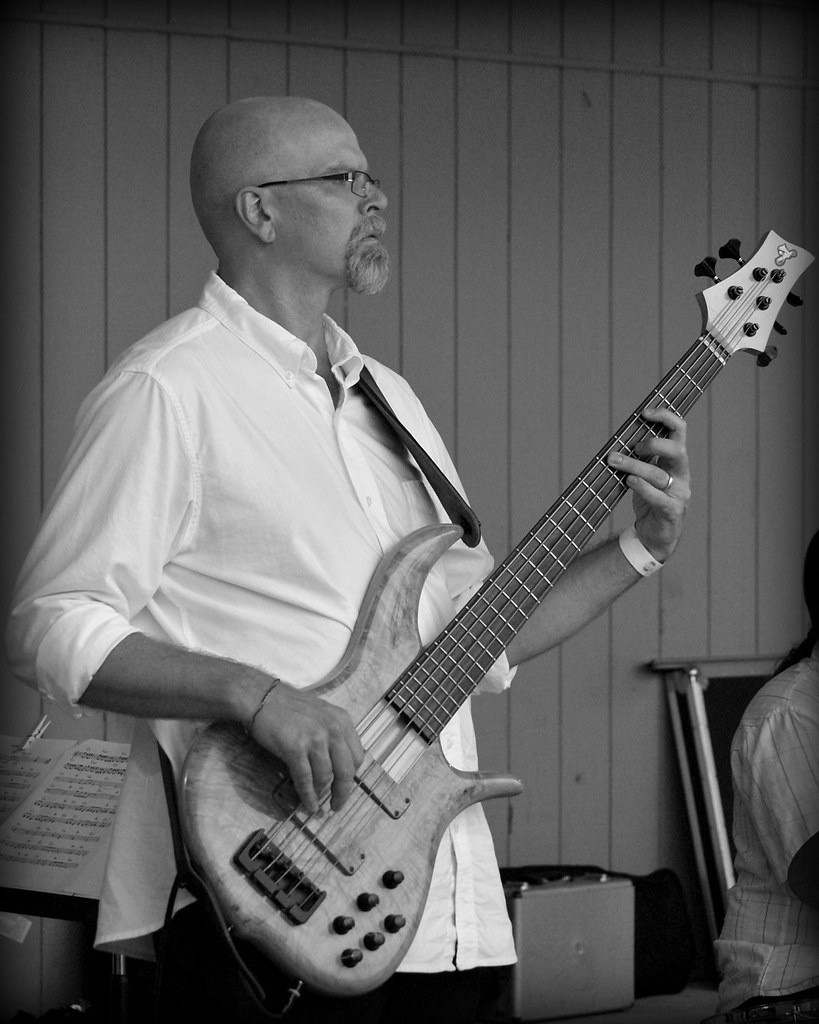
[710,522,818,1024]
[0,90,691,1023]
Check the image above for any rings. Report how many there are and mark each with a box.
[663,473,679,493]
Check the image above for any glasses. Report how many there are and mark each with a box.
[257,171,382,197]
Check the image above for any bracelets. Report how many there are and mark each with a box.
[231,679,288,743]
[615,525,669,581]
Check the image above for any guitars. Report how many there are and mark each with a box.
[177,222,815,995]
